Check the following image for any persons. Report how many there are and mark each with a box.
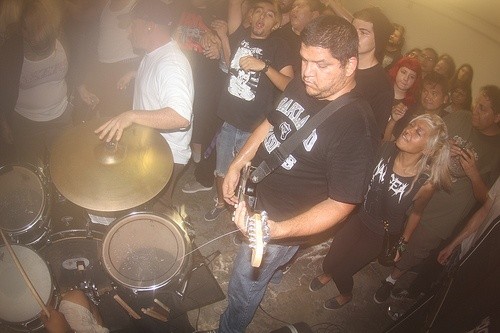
[193,13,373,332]
[0,0,500,332]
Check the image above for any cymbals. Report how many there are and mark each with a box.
[48,115,174,212]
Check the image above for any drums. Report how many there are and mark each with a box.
[0,161,52,246]
[36,192,104,292]
[102,212,186,291]
[1,243,58,330]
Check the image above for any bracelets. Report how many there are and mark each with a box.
[259,58,270,73]
[400,239,408,245]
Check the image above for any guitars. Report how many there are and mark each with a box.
[232,166,270,268]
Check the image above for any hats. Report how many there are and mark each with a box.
[116,0,175,27]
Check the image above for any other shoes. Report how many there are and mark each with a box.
[182,181,214,194]
[205,204,226,222]
[323,295,353,310]
[309,273,331,292]
[381,234,409,265]
[390,287,419,302]
[387,306,406,320]
[374,277,397,304]
